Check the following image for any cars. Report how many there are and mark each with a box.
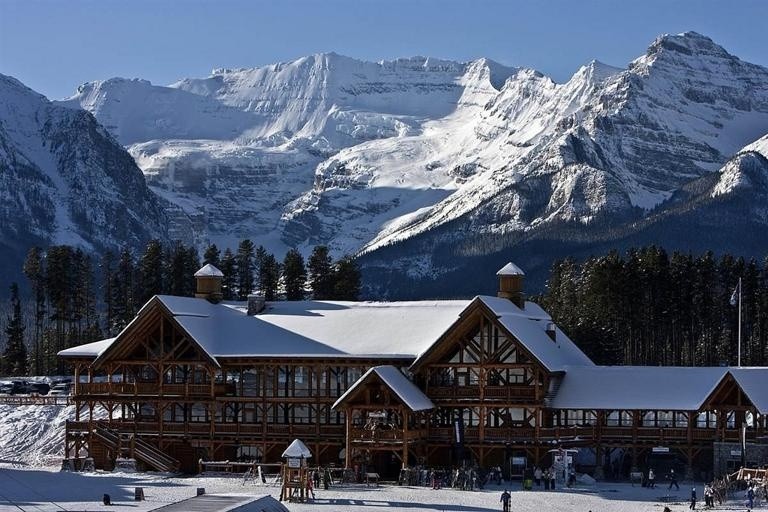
[0,376,121,397]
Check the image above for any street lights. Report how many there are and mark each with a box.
[730,276,746,410]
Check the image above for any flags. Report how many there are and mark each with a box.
[728,281,739,307]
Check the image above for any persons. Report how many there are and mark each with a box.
[387,467,578,492]
[305,466,333,498]
[499,488,510,512]
[645,466,768,512]
